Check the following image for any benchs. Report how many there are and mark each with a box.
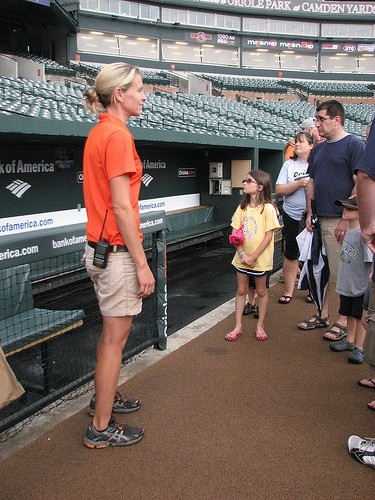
[0,205,232,295]
[0,264,86,421]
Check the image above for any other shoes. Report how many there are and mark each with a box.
[278,273,299,286]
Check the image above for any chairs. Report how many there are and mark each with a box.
[0,50,375,143]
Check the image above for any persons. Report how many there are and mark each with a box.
[225,170,280,342]
[83,62,155,449]
[286,99,375,469]
[275,131,317,304]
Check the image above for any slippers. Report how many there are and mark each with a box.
[254,329,268,341]
[225,330,242,341]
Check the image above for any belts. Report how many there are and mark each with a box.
[88,240,129,252]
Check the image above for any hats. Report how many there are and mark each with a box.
[334,194,357,210]
[301,118,315,130]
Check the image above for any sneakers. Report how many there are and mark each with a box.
[348,349,364,364]
[88,393,141,416]
[329,340,354,352]
[84,416,144,449]
[242,302,256,315]
[254,307,259,319]
[347,435,375,469]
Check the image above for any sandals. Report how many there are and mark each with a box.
[323,323,347,341]
[368,400,375,412]
[358,378,375,389]
[297,314,330,330]
[306,295,314,304]
[279,295,292,304]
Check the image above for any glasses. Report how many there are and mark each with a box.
[242,179,259,184]
